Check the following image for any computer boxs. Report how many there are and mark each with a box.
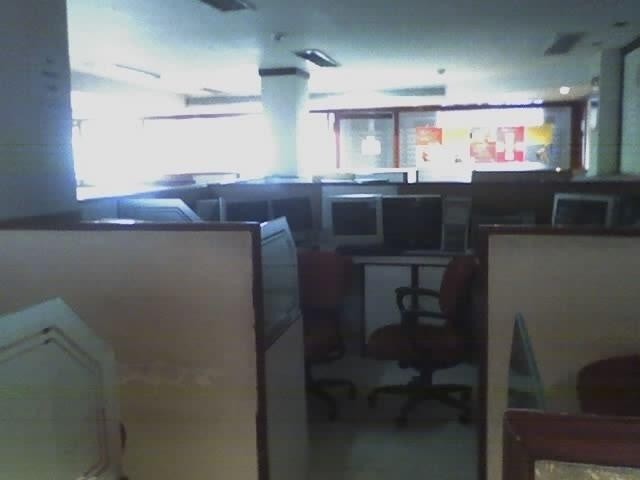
[380,194,446,251]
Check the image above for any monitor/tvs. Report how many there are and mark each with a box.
[220,198,269,223]
[270,196,316,241]
[552,194,613,230]
[326,194,384,247]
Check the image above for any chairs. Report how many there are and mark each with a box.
[369,255,487,431]
[297,249,353,421]
[577,353,640,418]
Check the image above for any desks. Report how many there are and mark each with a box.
[353,250,454,345]
